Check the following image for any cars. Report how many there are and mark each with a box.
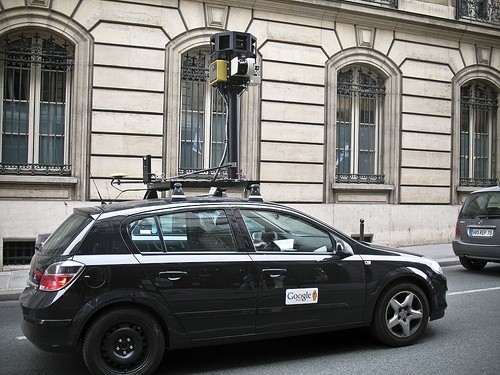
[452,185,500,270]
[18,154,448,375]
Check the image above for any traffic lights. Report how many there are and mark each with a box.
[209,60,226,87]
